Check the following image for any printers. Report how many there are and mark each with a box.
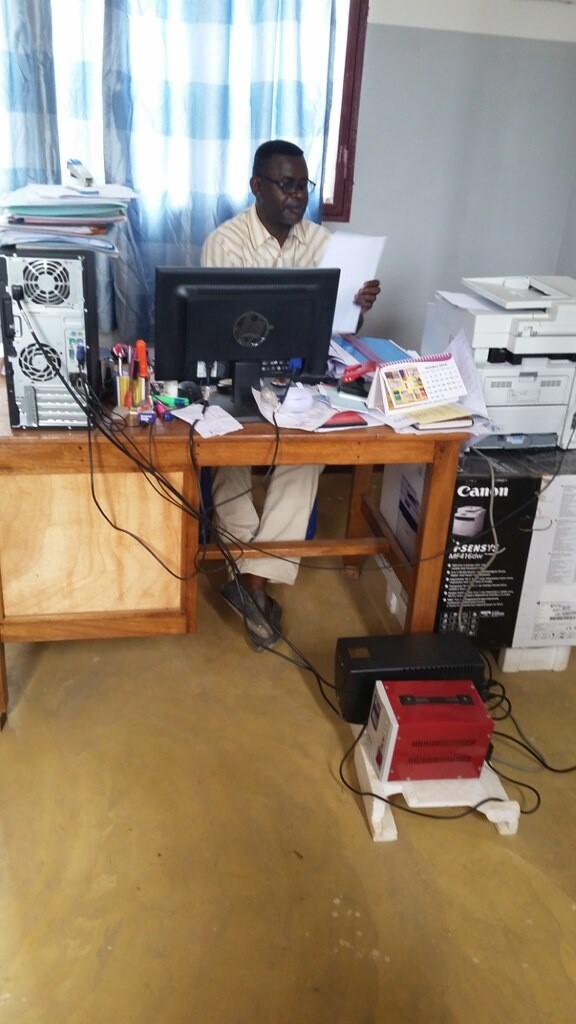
[420,274,576,451]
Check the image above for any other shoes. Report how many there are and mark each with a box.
[219,580,274,639]
[246,598,282,653]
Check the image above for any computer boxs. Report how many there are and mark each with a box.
[0,244,120,430]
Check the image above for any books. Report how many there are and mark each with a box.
[0,183,139,257]
[412,415,475,430]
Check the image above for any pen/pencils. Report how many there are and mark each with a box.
[118,344,138,379]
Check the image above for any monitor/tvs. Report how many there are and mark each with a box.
[154,268,341,423]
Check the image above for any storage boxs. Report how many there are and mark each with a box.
[371,450,576,648]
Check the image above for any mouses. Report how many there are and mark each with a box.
[176,380,204,404]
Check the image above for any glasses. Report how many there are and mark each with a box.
[257,172,316,197]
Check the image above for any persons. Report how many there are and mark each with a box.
[199,140,381,650]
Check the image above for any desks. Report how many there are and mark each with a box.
[0,335,473,730]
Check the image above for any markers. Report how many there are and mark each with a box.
[151,393,189,421]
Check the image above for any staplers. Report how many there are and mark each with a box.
[61,158,99,194]
